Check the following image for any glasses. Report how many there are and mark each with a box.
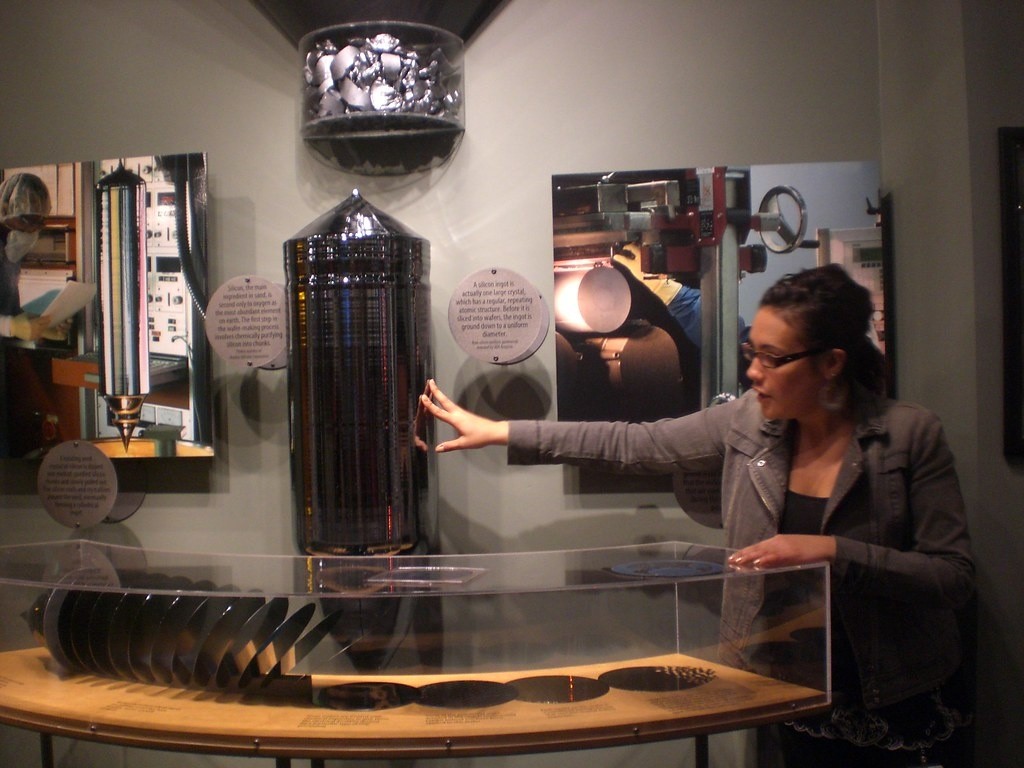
[741,342,826,369]
[18,215,44,229]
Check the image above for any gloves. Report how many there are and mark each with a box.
[613,245,683,307]
[9,312,51,342]
[43,317,72,341]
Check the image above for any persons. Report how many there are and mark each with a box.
[0,173,74,459]
[613,245,753,394]
[418,263,979,768]
